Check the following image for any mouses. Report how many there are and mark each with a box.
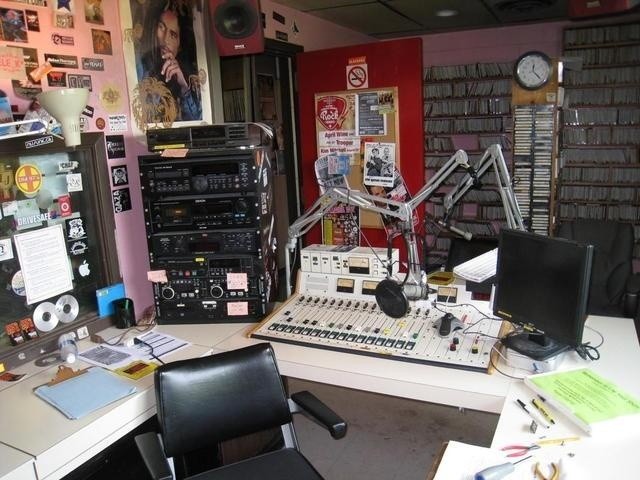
[431,312,464,338]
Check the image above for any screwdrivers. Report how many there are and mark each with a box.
[474,455,532,480]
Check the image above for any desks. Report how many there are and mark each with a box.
[486,312,640,479]
[0,310,270,480]
[214,310,522,422]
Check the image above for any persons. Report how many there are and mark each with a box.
[136,0,202,122]
[366,148,393,178]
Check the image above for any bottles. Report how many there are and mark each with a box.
[58,334,78,364]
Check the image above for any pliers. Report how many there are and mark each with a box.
[501,445,540,457]
[534,462,558,480]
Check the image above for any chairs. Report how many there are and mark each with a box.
[135,336,350,480]
[556,217,639,343]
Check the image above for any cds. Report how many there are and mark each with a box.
[54,293,81,325]
[32,301,58,333]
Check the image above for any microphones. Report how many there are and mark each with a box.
[399,283,428,299]
[436,216,475,244]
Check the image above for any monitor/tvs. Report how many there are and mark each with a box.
[490,226,595,363]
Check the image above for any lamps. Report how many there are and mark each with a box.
[0,84,91,150]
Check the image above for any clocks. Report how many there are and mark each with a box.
[515,49,553,89]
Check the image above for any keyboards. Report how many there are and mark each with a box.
[452,245,499,284]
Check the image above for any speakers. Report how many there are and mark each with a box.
[208,0,266,59]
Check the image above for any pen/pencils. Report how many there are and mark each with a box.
[125,299,129,310]
[517,399,555,429]
[536,437,579,445]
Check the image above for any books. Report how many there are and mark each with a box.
[423,23,639,265]
[524,365,640,435]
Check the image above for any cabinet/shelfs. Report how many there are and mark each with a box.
[422,73,511,271]
[561,18,640,248]
